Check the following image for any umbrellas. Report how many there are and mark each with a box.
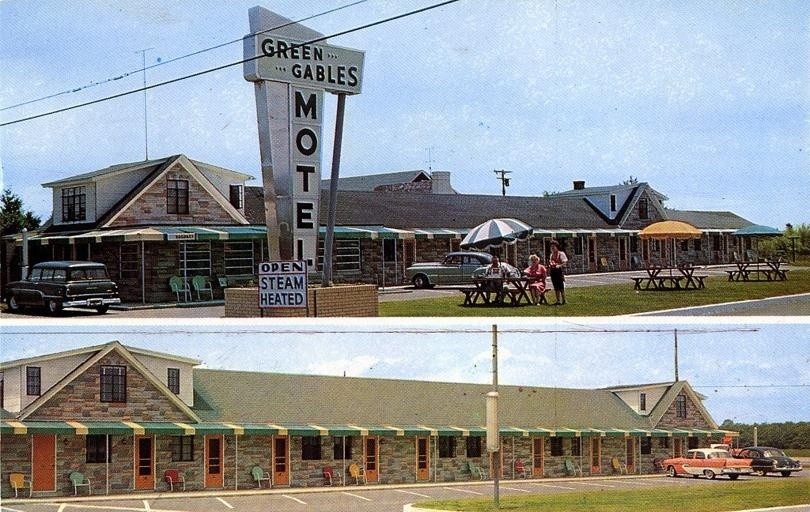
[458,218,534,263]
[639,220,704,267]
[730,224,784,262]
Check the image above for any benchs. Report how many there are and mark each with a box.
[724,269,789,282]
[656,275,708,290]
[503,288,551,307]
[459,287,519,307]
[630,276,684,290]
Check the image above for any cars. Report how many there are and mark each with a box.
[729,448,750,455]
[663,447,755,481]
[733,447,802,478]
[711,443,736,456]
[404,251,522,289]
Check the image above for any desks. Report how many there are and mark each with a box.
[645,266,701,290]
[473,277,529,305]
[731,261,784,280]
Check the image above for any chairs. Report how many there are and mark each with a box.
[468,459,487,482]
[601,256,614,270]
[251,465,272,490]
[564,459,581,477]
[168,276,213,301]
[69,471,91,495]
[515,458,533,479]
[733,251,741,263]
[9,474,34,498]
[611,455,627,475]
[654,458,662,473]
[322,464,367,487]
[164,470,185,490]
[633,255,645,268]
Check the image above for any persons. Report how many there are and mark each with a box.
[523,253,547,306]
[485,255,510,293]
[548,239,569,305]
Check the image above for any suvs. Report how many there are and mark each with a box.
[4,262,121,316]
